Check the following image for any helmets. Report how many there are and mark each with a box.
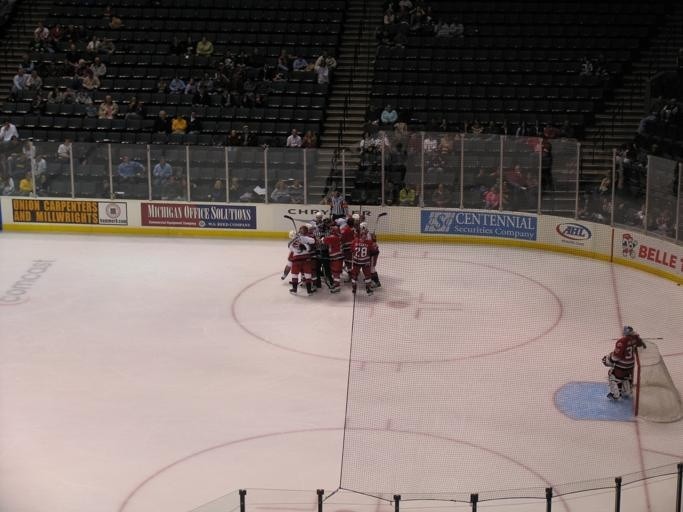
[623,326,633,336]
[288,211,368,240]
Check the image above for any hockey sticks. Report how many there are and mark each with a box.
[373,213,387,235]
[284,215,297,235]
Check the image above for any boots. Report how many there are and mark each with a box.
[281,274,381,295]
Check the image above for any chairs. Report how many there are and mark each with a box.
[0,0,631,183]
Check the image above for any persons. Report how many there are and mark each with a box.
[602,324,646,400]
